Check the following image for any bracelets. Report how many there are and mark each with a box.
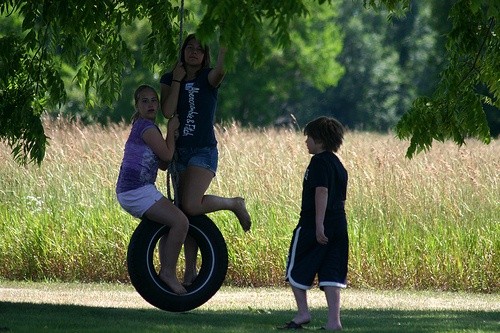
[172,79,181,85]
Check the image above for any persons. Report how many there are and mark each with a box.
[116,86,190,295]
[158,33,252,287]
[276,117,349,332]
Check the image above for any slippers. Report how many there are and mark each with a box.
[316,326,326,330]
[277,320,310,331]
